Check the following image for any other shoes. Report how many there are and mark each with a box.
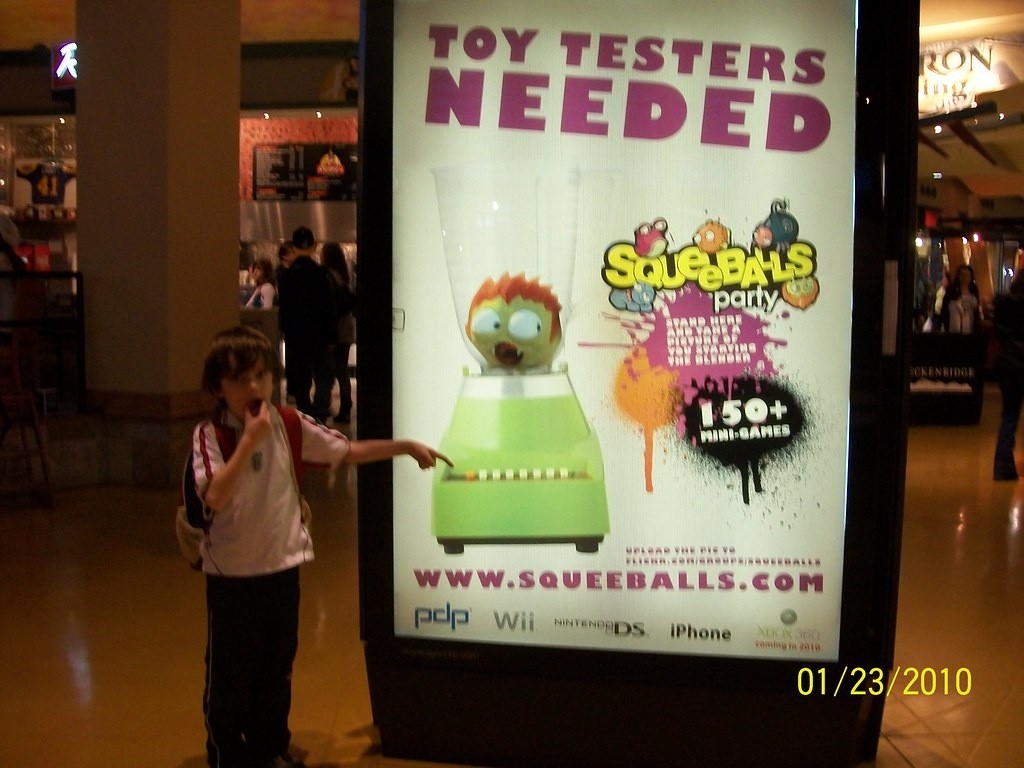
[270,751,306,768]
[993,452,1018,481]
[333,406,352,423]
[302,410,327,428]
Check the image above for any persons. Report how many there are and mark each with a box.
[0,210,26,346]
[278,225,343,429]
[990,264,1024,480]
[276,240,301,406]
[246,257,288,381]
[913,263,982,335]
[189,323,454,768]
[315,238,357,425]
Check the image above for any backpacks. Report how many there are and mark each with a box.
[977,313,1006,388]
[175,402,313,571]
